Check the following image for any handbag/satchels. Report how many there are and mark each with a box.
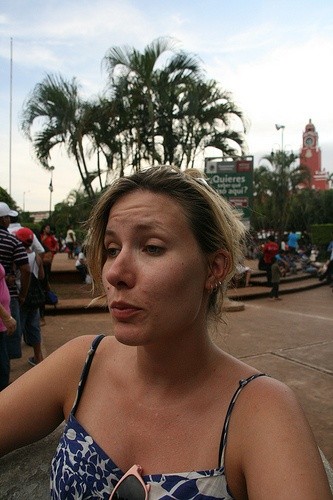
[46,290,58,305]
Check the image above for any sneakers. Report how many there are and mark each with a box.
[28,357,38,366]
[9,353,21,359]
[40,321,45,326]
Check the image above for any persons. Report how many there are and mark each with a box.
[0,164,333,500]
[0,195,333,391]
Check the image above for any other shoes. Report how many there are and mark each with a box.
[282,271,286,277]
[266,297,274,301]
[275,298,282,300]
[266,283,273,287]
[245,283,253,288]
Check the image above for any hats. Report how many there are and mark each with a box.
[12,228,34,246]
[0,202,18,217]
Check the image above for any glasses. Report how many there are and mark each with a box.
[108,465,150,500]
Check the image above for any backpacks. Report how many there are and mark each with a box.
[17,251,46,311]
